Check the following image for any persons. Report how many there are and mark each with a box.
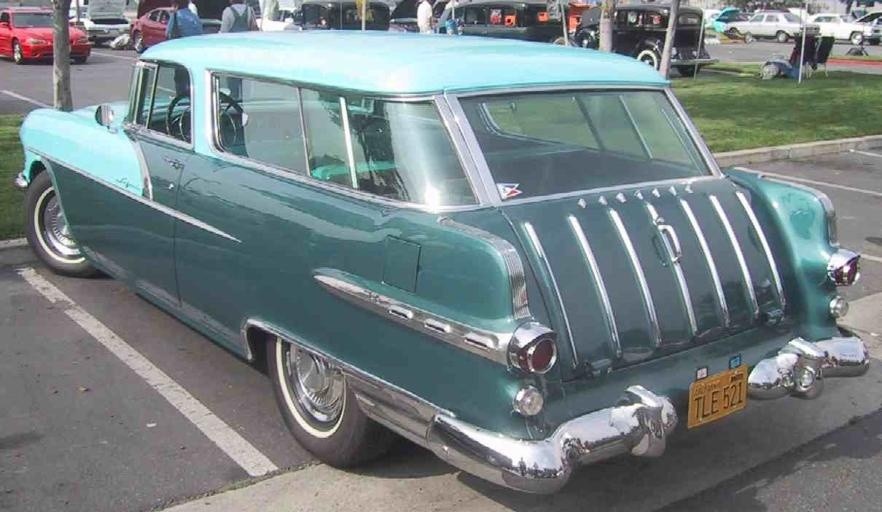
[165,1,205,106]
[217,1,261,104]
[416,0,437,34]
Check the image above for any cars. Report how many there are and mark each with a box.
[16,28,869,494]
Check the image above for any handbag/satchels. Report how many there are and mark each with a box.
[168,13,180,38]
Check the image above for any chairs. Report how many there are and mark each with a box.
[760,35,835,79]
